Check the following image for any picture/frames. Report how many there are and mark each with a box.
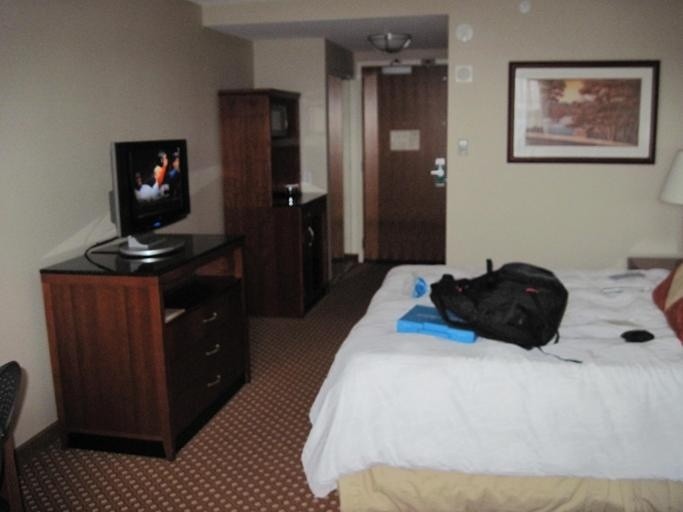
[506,59,661,167]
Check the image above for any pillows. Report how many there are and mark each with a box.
[652,268,681,344]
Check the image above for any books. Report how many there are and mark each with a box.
[390,303,479,343]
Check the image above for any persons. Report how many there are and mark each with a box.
[129,147,183,209]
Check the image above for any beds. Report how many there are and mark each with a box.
[335,264,681,511]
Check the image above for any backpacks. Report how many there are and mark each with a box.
[430,258,569,350]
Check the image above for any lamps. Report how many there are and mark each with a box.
[367,30,411,54]
[657,148,682,263]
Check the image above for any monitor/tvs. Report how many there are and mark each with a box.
[108,139,191,258]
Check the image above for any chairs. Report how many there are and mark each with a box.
[0,360,33,509]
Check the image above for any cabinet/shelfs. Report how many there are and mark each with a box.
[38,234,255,462]
[217,87,304,204]
[221,192,329,321]
